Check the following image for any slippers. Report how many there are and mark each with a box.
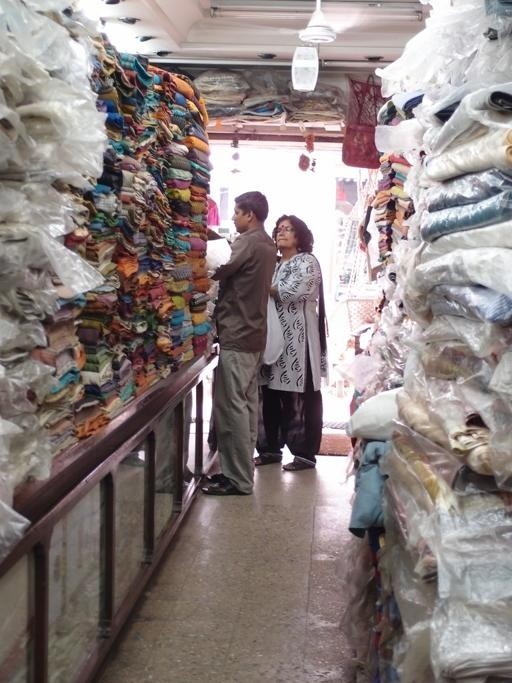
[252,453,282,466]
[282,461,315,470]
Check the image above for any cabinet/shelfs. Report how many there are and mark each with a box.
[0,355,218,683]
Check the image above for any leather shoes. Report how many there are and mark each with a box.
[201,471,253,495]
[155,471,194,494]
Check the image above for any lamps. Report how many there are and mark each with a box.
[290,0,337,93]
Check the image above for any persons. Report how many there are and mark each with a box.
[251,212,331,471]
[200,190,277,493]
